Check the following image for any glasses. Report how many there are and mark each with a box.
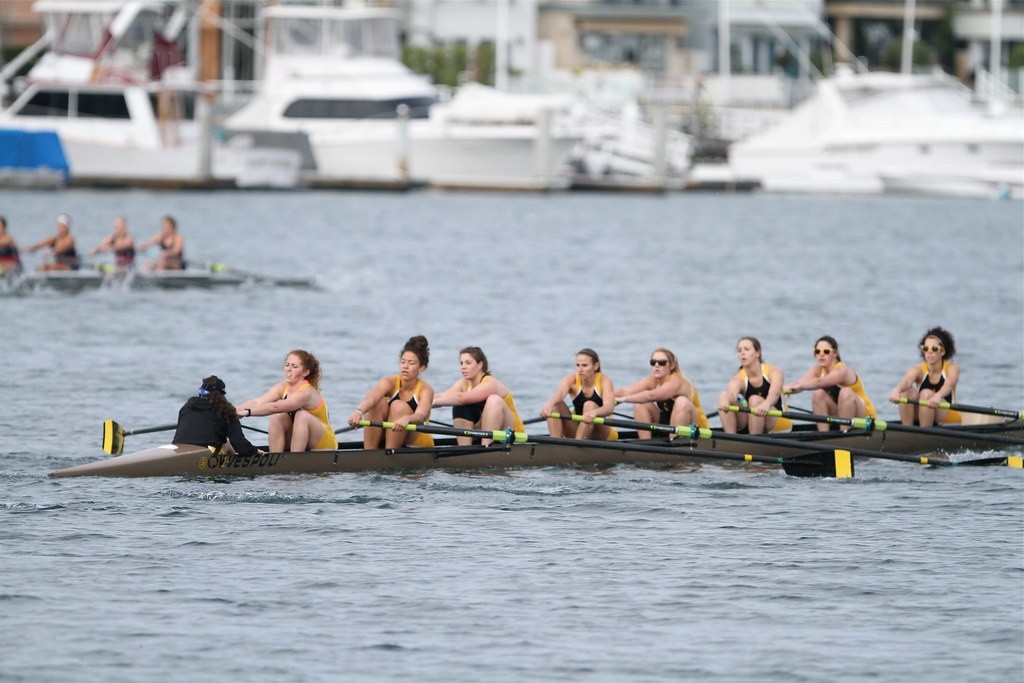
[814,349,837,354]
[650,359,669,366]
[920,345,944,352]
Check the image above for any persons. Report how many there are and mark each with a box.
[615,348,708,439]
[717,336,792,434]
[172,375,263,454]
[890,327,961,427]
[237,350,338,452]
[0,219,20,276]
[348,335,433,449]
[139,217,183,270]
[540,349,617,440]
[28,214,76,270]
[92,219,134,272]
[785,336,876,432]
[431,347,524,445]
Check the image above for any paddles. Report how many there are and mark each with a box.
[170,256,315,288]
[100,419,177,458]
[551,412,1023,469]
[357,418,855,479]
[900,397,1023,420]
[522,400,621,424]
[704,389,796,418]
[728,404,1023,445]
[335,423,361,434]
[52,255,120,276]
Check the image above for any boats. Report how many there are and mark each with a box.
[1,0,1024,195]
[44,407,1024,481]
[16,260,319,289]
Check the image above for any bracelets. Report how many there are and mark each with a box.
[355,409,364,414]
[245,409,251,417]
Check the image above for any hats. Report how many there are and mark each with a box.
[56,214,71,225]
